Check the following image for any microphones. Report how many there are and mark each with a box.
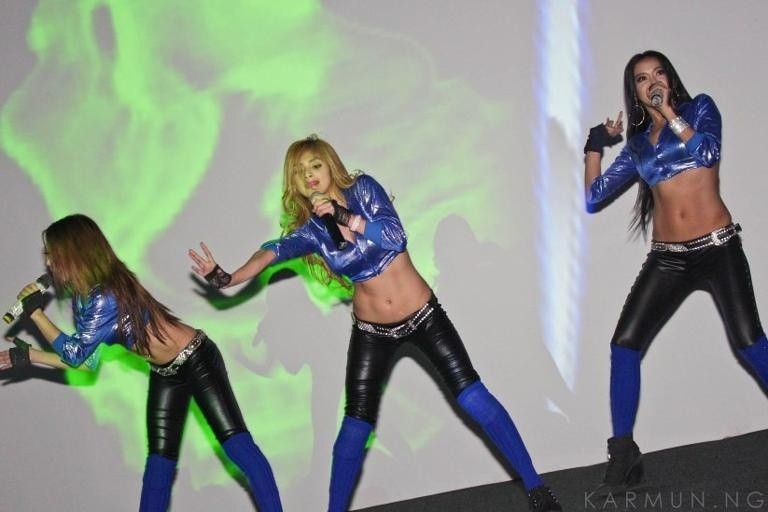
[309,189,347,251]
[3,272,53,324]
[648,83,663,107]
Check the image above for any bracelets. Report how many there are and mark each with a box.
[667,116,691,137]
[348,214,362,233]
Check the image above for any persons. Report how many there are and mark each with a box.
[186,131,566,510]
[583,50,768,499]
[0,212,287,512]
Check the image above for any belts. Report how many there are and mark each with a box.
[649,221,742,254]
[349,300,435,341]
[145,329,206,379]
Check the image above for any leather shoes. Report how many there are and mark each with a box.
[527,483,563,512]
[597,433,644,499]
